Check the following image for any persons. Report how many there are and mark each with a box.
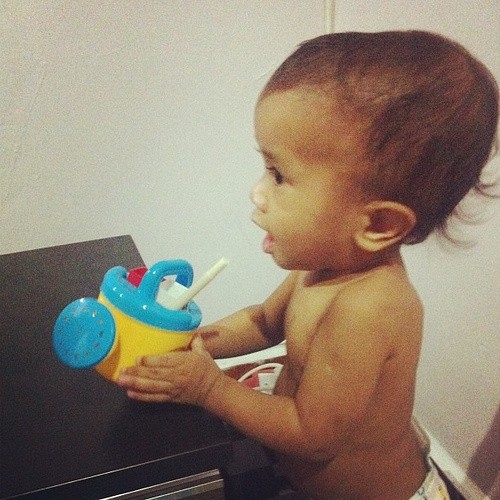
[114,29,500,500]
